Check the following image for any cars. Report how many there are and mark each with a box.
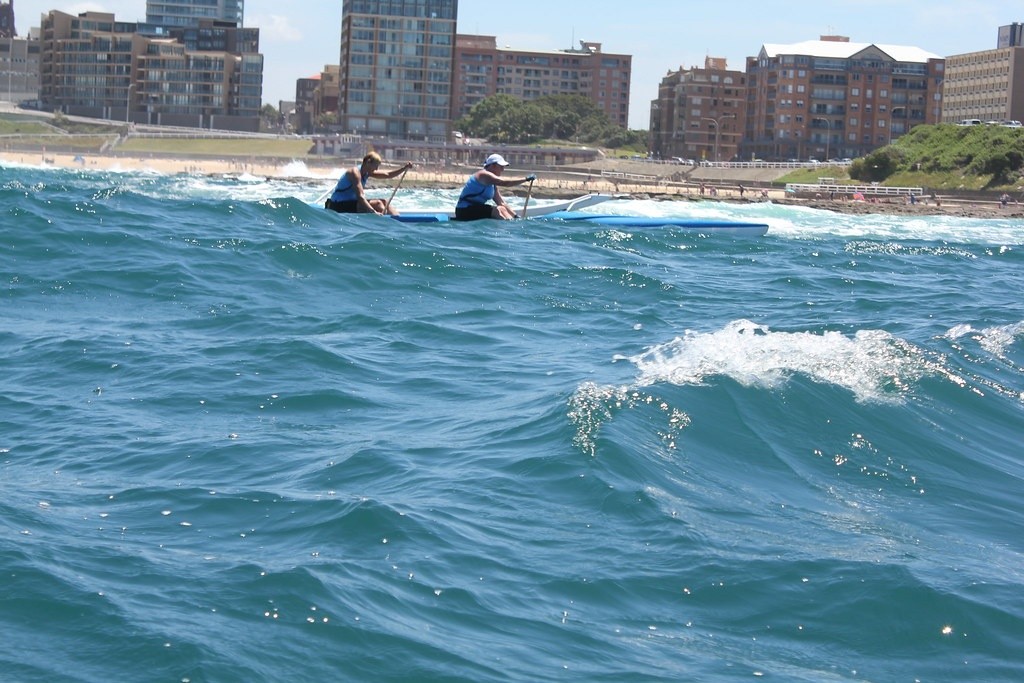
[961,119,1022,126]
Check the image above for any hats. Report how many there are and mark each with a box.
[484,153,510,169]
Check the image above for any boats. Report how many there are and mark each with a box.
[381,210,771,238]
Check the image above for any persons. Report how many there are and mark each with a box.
[567,180,1023,206]
[454,153,535,220]
[325,152,412,216]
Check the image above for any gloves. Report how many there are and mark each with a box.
[526,174,535,181]
[514,214,520,218]
